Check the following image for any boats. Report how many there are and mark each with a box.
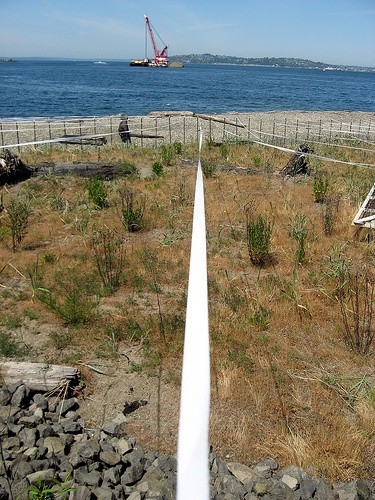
[129,15,169,67]
[59,134,107,146]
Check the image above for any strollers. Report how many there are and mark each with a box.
[275,139,314,182]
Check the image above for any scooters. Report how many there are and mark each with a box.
[118,120,130,142]
